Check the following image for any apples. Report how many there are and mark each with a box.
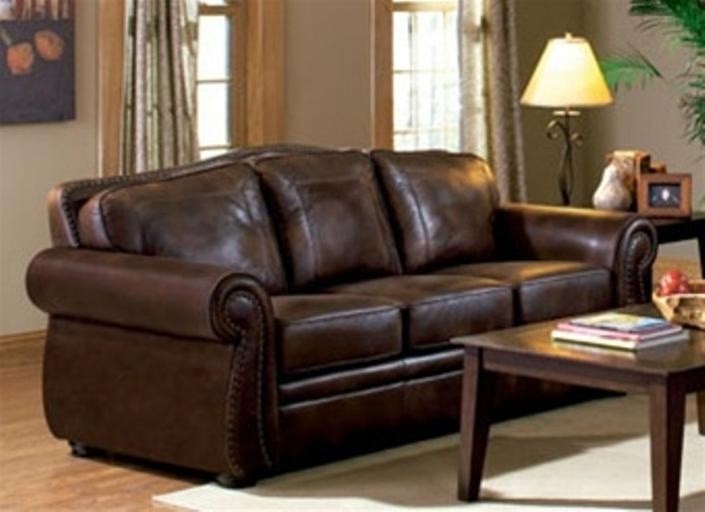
[657,268,694,295]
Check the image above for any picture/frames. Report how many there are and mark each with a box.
[634,173,693,220]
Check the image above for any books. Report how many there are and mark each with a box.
[549,326,691,352]
[556,311,684,342]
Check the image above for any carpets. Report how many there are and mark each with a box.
[153,395,705,512]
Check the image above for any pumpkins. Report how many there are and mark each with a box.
[593,159,633,211]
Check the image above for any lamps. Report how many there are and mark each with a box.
[518,29,614,204]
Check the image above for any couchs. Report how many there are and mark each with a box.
[25,143,659,489]
